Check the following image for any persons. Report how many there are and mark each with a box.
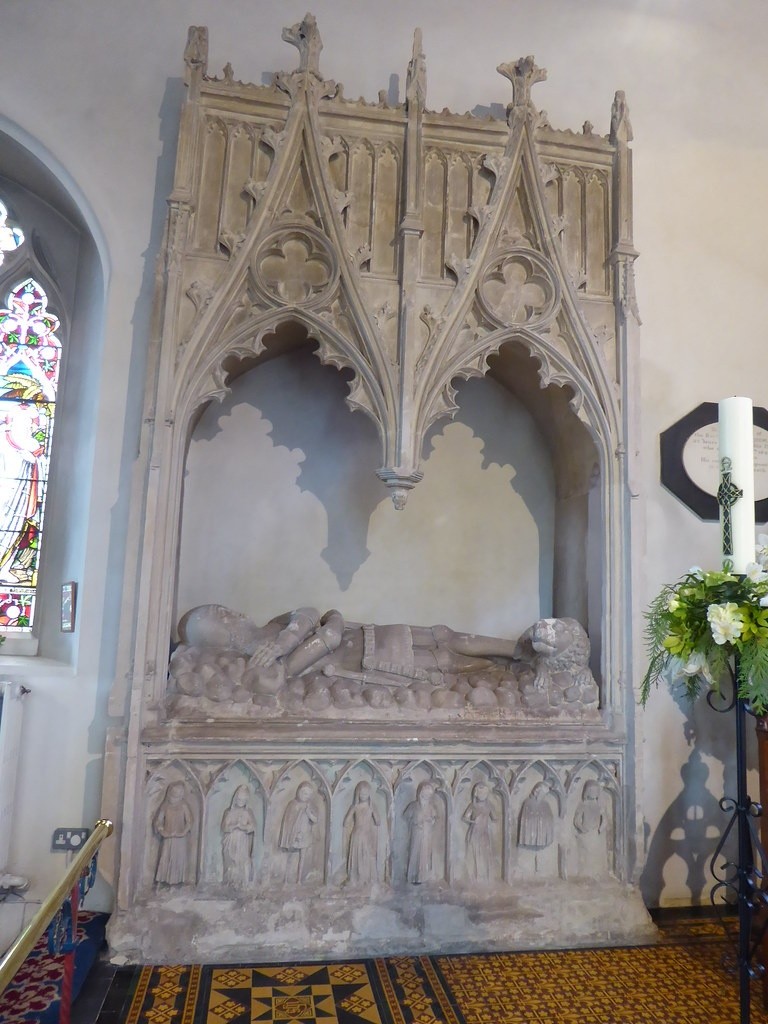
[573,780,610,875]
[343,782,381,881]
[462,782,499,881]
[278,782,319,883]
[221,785,256,881]
[517,783,554,846]
[178,604,540,679]
[152,782,193,885]
[404,782,438,883]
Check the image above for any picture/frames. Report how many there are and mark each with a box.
[61,581,75,632]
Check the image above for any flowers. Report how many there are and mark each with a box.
[635,562,768,717]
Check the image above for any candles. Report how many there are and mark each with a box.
[718,396,756,575]
